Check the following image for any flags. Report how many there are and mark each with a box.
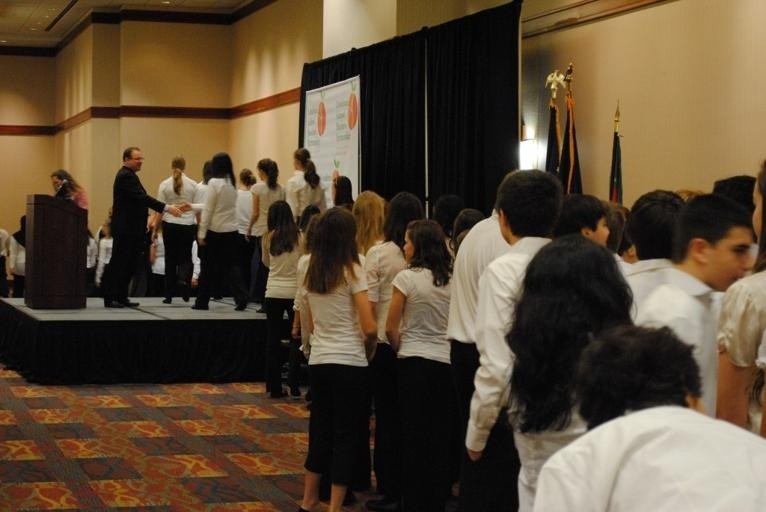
[609,128,624,207]
[544,99,560,173]
[557,94,585,193]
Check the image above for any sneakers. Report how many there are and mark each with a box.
[163,297,173,304]
[191,304,209,310]
[287,382,302,396]
[266,385,289,399]
[182,293,190,301]
[235,304,246,310]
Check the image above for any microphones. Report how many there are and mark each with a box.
[54,179,68,198]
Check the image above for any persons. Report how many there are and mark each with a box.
[102,146,182,307]
[87,205,224,300]
[150,154,198,304]
[236,167,256,295]
[0,227,9,298]
[285,148,326,227]
[178,152,251,310]
[260,163,766,512]
[9,215,25,297]
[244,158,285,313]
[196,160,212,255]
[50,169,87,209]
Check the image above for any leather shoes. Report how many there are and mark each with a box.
[105,300,123,308]
[120,299,140,307]
